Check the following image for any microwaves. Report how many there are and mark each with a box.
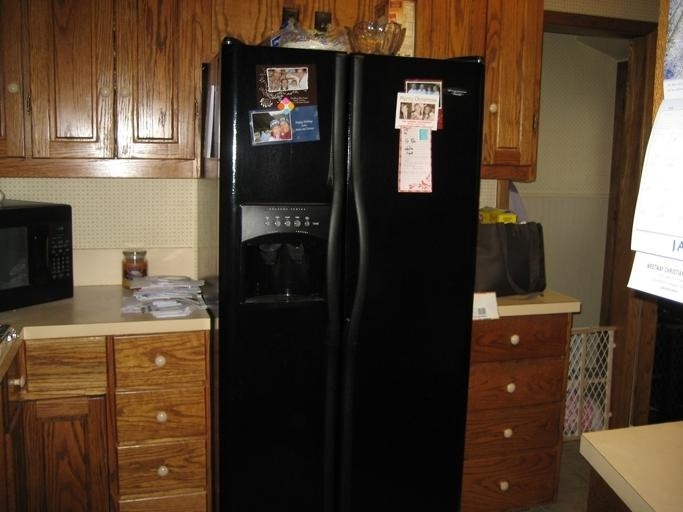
[0,199,74,313]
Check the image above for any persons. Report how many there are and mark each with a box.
[409,104,423,120]
[425,85,433,96]
[400,104,410,119]
[417,84,426,94]
[432,86,439,96]
[407,84,417,94]
[422,105,435,121]
[266,119,281,142]
[269,69,308,91]
[278,117,290,140]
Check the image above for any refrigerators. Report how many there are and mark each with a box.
[197,35,486,512]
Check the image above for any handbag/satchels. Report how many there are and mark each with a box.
[475,222,546,296]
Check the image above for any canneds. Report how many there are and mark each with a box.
[121,250,147,290]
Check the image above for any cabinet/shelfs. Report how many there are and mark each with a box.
[460,313,572,512]
[0,0,544,184]
[0,336,118,512]
[112,331,211,511]
